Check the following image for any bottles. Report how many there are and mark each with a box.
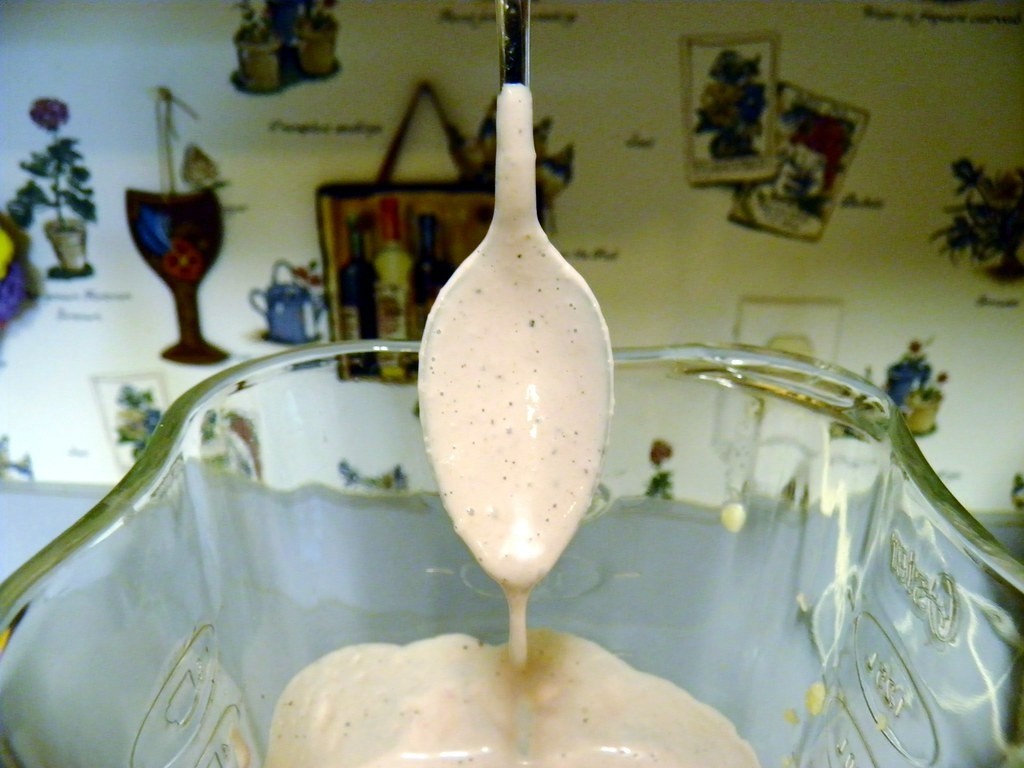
[335,197,452,381]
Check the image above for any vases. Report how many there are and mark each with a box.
[45,219,86,274]
[294,13,338,75]
[235,27,281,92]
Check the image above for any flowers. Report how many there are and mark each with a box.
[296,0,338,31]
[234,0,273,44]
[4,97,102,229]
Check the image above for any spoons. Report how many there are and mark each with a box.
[416,1,615,586]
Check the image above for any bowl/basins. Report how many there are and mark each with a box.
[0,335,1024,768]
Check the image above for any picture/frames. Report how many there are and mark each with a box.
[679,31,869,241]
